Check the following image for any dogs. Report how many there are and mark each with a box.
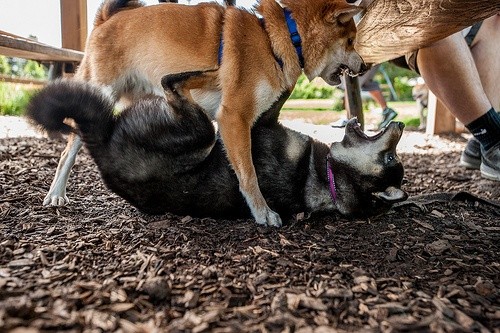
[41,0,367,227]
[23,68,409,218]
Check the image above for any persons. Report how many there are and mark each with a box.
[403,10,500,181]
[329,63,397,129]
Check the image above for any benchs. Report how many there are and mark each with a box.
[0,31,85,85]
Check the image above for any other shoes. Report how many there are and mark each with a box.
[461,138,481,168]
[378,108,397,129]
[330,118,347,127]
[480,144,500,181]
